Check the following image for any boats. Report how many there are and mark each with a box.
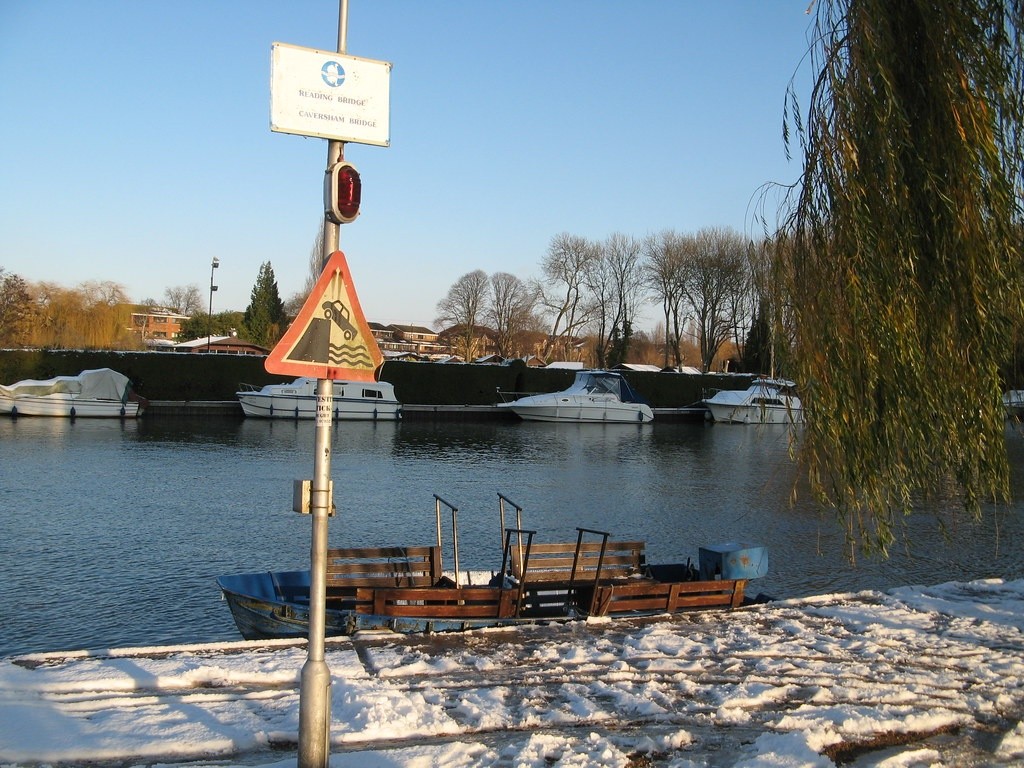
[508,369,654,424]
[701,377,816,429]
[238,375,402,431]
[0,368,149,422]
[217,485,772,654]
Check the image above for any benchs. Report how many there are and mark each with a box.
[584,578,747,618]
[353,588,524,625]
[327,546,443,589]
[511,542,647,591]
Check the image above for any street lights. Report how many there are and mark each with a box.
[204,256,220,355]
